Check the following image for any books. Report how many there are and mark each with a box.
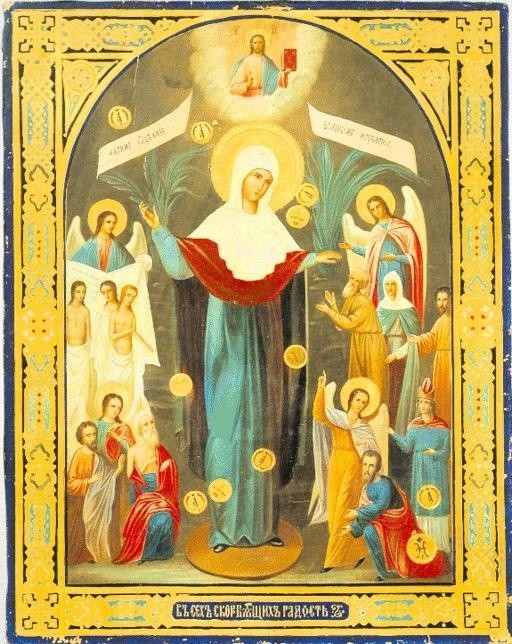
[282,47,299,73]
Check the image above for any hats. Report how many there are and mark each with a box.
[417,377,436,400]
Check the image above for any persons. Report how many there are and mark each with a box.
[229,32,292,99]
[307,183,456,582]
[136,143,346,556]
